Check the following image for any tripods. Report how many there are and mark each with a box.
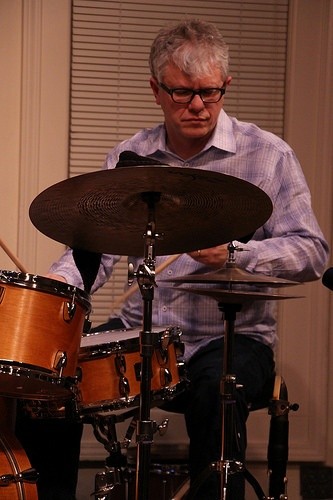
[170,296,268,500]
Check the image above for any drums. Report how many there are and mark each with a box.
[0,432,39,500]
[1,270,93,401]
[21,325,187,421]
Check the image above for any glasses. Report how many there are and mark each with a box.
[157,80,226,104]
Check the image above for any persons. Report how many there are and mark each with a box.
[43,17,329,500]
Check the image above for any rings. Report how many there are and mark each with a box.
[198,250,201,257]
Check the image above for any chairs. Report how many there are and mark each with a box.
[156,375,289,500]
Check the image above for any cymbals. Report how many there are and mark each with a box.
[29,167,273,257]
[155,268,300,284]
[161,286,307,304]
